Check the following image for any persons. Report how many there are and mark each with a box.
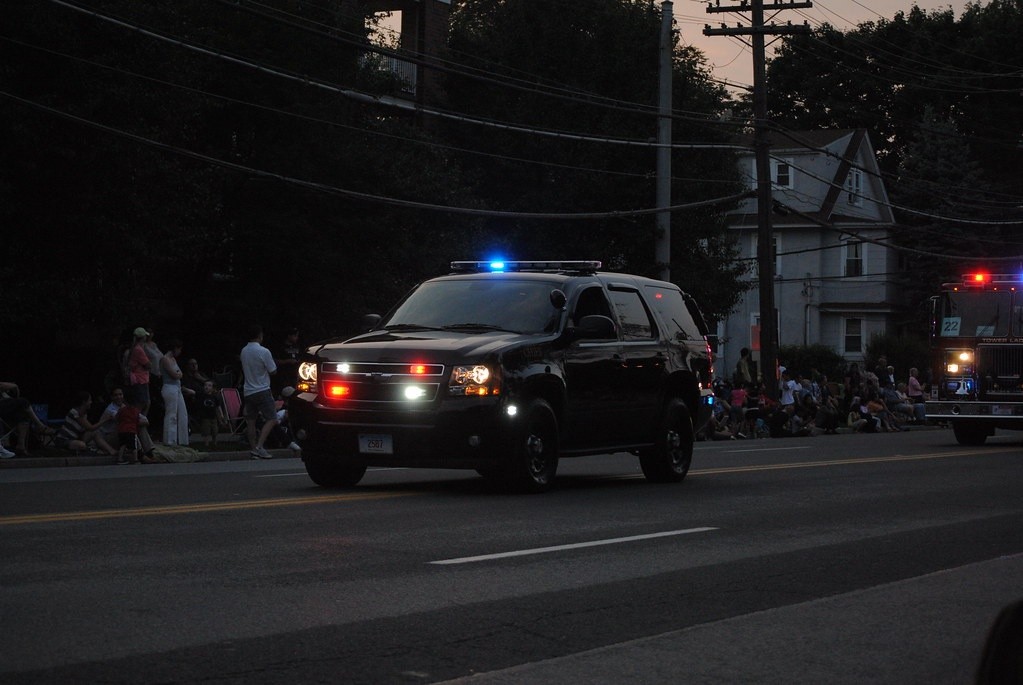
[0,317,308,465]
[695,347,950,442]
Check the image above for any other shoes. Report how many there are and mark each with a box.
[152,455,167,463]
[116,460,130,465]
[202,445,210,452]
[134,460,142,464]
[37,426,56,436]
[722,418,928,441]
[288,442,301,451]
[212,446,221,451]
[16,446,34,457]
[141,455,157,464]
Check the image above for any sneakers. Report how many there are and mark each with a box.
[0,444,15,459]
[250,446,272,459]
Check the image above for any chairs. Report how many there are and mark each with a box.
[220,388,250,447]
[0,399,63,455]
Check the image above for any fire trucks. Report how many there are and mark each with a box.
[922,271,1023,444]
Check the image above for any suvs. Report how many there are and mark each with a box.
[288,258,714,491]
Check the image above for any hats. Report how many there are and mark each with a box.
[133,327,150,337]
[288,327,301,335]
